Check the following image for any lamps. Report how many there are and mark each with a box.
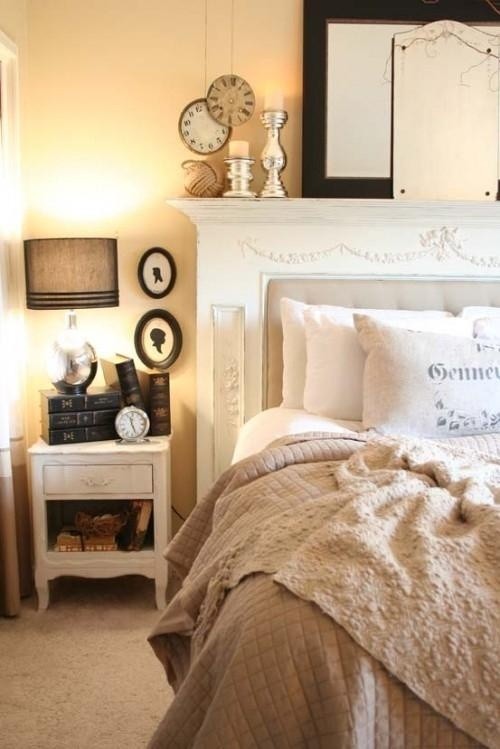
[22,235,121,394]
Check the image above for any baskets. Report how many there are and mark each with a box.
[75,507,130,535]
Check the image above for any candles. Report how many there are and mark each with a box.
[227,139,250,158]
[263,96,285,111]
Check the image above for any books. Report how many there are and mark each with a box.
[55,499,153,552]
[98,352,151,437]
[39,384,121,445]
[135,366,172,437]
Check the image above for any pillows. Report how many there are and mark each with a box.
[276,293,500,442]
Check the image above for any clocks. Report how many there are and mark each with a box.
[177,73,256,157]
[114,403,152,444]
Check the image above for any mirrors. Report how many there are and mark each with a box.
[303,1,500,196]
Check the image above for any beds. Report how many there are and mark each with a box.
[169,197,500,749]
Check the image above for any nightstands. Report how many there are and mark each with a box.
[26,430,173,615]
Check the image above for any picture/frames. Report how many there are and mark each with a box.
[133,246,183,373]
